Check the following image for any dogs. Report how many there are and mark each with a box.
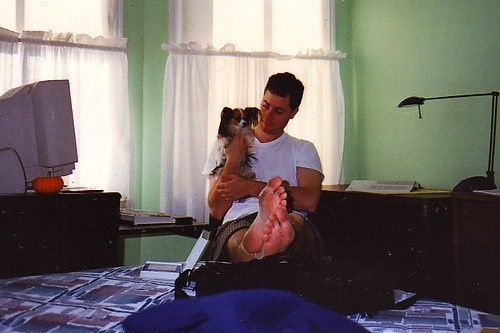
[208,105,262,202]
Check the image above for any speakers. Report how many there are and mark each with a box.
[0,148,26,194]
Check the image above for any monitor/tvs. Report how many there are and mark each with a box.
[0,80,78,195]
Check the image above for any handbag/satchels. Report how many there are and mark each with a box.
[174,252,338,308]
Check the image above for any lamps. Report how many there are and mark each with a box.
[397,91,500,192]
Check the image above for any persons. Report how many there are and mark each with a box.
[203,71,325,264]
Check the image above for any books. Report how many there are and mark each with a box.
[345,178,427,193]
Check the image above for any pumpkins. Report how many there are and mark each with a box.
[32,170,63,193]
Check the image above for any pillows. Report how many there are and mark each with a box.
[123,287,370,333]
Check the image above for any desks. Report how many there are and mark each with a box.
[120,222,210,268]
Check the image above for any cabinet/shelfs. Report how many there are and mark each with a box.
[0,189,121,278]
[451,191,500,315]
[311,184,452,296]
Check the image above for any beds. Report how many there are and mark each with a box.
[0,263,500,333]
